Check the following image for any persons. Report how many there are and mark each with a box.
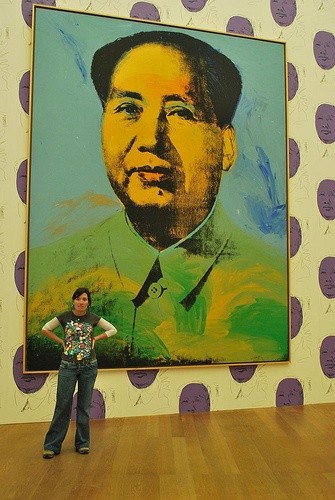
[42,287,117,459]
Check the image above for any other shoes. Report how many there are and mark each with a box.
[43,449,54,458]
[78,447,90,454]
